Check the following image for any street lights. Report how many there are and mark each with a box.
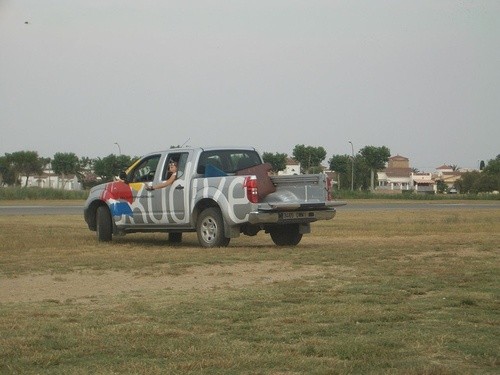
[349,141,354,190]
[114,142,123,155]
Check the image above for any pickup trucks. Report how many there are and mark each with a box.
[84,148,337,249]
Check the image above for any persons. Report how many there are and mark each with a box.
[145,155,184,191]
